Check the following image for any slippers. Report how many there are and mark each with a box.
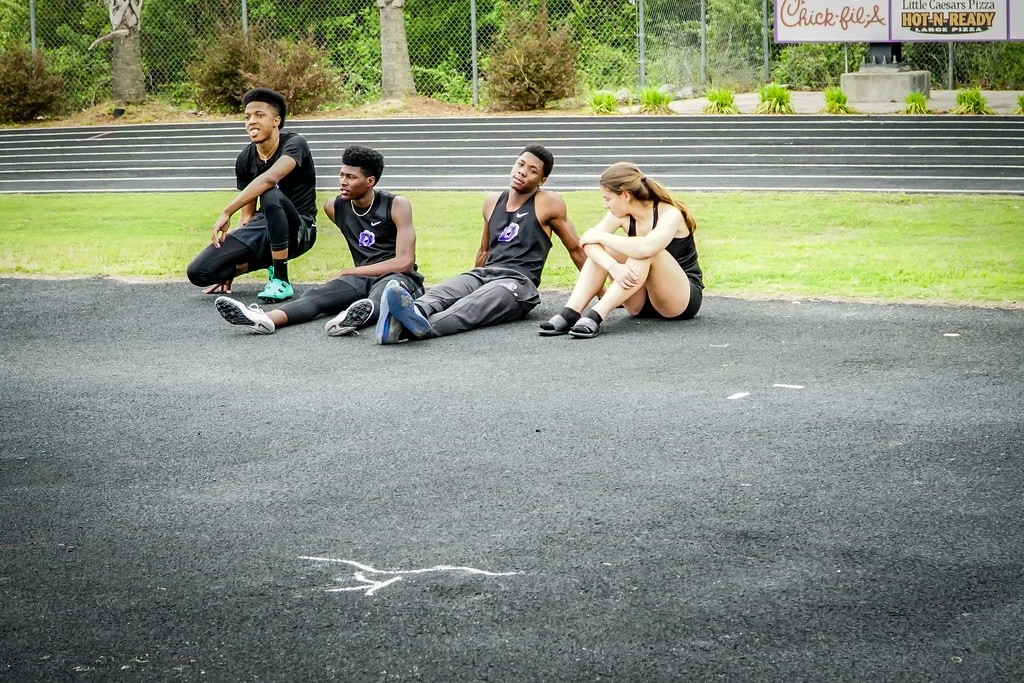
[538,314,570,334]
[568,317,605,337]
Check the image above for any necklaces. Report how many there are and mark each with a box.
[256,138,279,163]
[351,196,375,217]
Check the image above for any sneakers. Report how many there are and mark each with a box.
[325,298,374,336]
[387,285,432,339]
[265,266,274,290]
[258,279,294,303]
[215,296,275,334]
[375,279,409,344]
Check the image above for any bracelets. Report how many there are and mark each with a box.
[606,261,619,274]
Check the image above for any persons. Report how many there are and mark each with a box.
[375,143,624,345]
[539,162,705,338]
[187,88,318,303]
[213,146,425,337]
